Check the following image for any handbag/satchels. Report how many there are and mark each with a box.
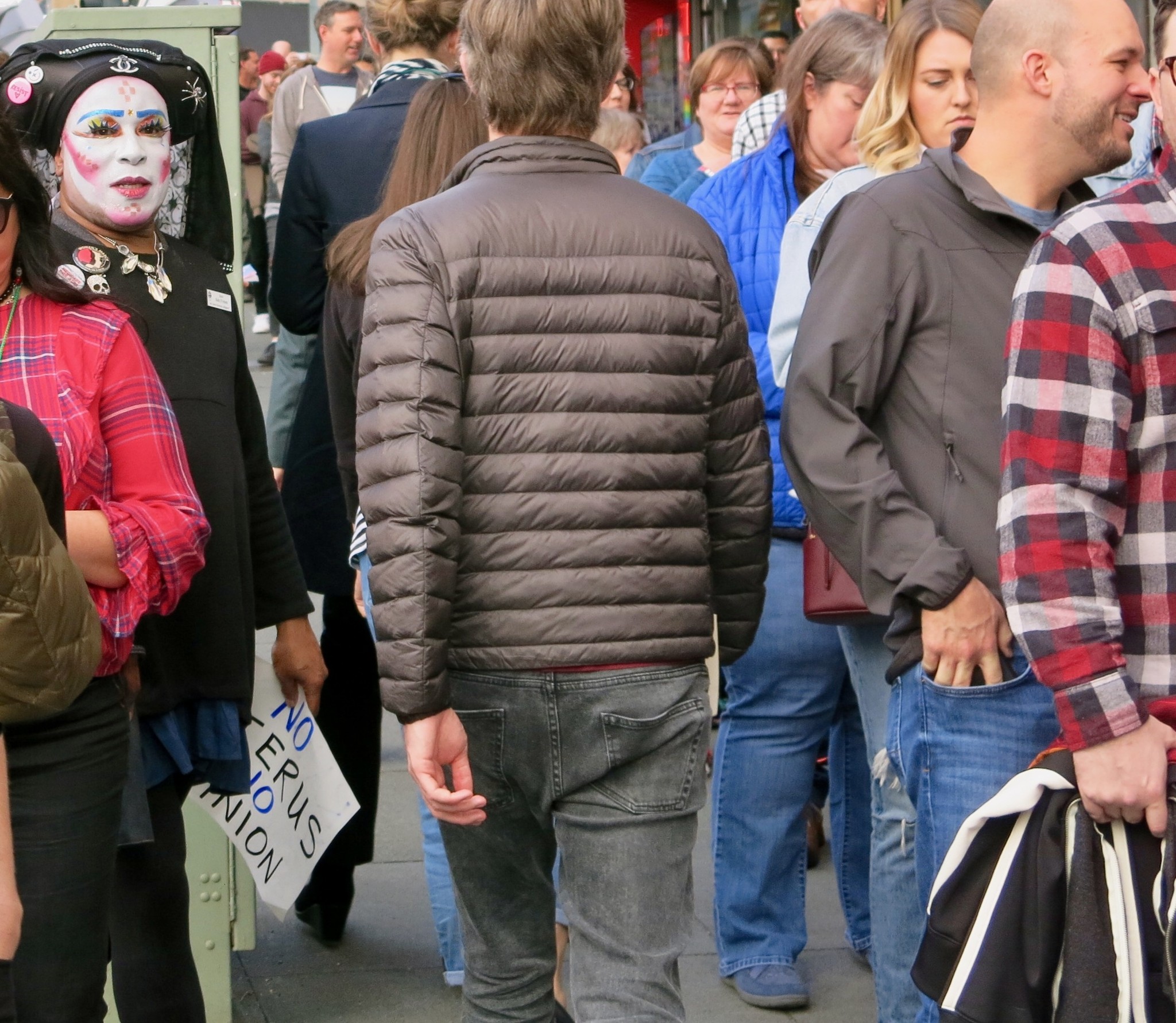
[800,517,878,629]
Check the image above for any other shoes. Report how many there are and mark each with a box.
[726,964,812,1011]
[252,312,271,334]
[257,343,276,367]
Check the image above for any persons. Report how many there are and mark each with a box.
[355,0,771,1023]
[0,0,1176,1023]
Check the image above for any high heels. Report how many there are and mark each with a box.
[296,877,356,943]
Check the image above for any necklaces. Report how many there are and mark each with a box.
[0,286,21,363]
[0,268,23,303]
[88,224,172,304]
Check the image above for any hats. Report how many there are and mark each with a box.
[258,51,285,74]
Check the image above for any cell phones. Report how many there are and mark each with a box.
[964,646,1019,687]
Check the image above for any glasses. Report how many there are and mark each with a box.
[704,81,761,101]
[614,76,633,91]
